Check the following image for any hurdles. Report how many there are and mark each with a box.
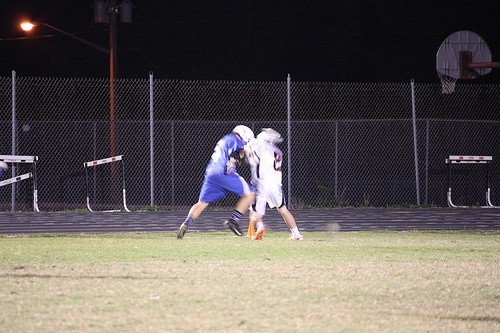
[0,154,43,212]
[443,156,497,209]
[83,155,133,212]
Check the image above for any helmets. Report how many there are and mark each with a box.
[258,128,282,144]
[233,124,254,143]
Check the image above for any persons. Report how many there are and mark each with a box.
[176,124,305,243]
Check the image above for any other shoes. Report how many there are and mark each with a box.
[176,222,188,238]
[288,234,303,242]
[253,227,265,240]
[224,218,243,237]
